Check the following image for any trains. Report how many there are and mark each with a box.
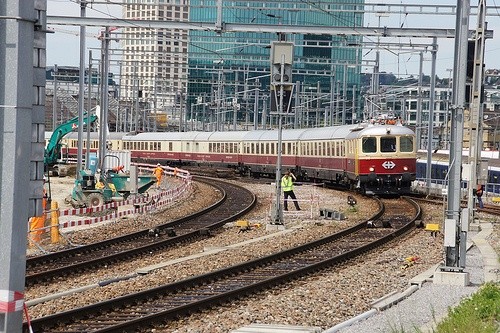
[43,114,419,199]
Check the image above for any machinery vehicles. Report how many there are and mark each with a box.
[70,155,162,209]
[43,105,109,180]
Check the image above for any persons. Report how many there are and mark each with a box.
[151,163,164,190]
[280,169,303,212]
[473,183,488,210]
